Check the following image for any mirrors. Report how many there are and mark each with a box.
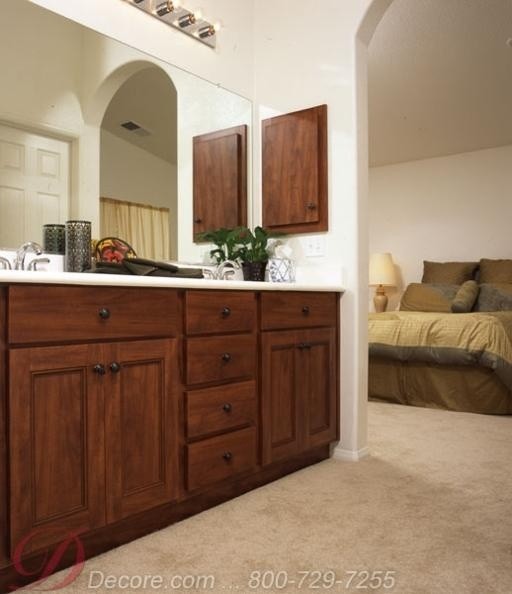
[0,0,253,266]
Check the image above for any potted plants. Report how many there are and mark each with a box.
[231,226,272,281]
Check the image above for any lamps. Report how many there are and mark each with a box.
[369,252,398,313]
[130,0,216,39]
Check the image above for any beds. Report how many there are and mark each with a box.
[366,312,512,415]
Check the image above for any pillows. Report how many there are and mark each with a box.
[400,259,511,313]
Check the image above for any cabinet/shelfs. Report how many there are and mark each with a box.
[193,125,246,241]
[261,105,329,233]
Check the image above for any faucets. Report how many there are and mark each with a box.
[215,260,240,280]
[13,241,41,270]
